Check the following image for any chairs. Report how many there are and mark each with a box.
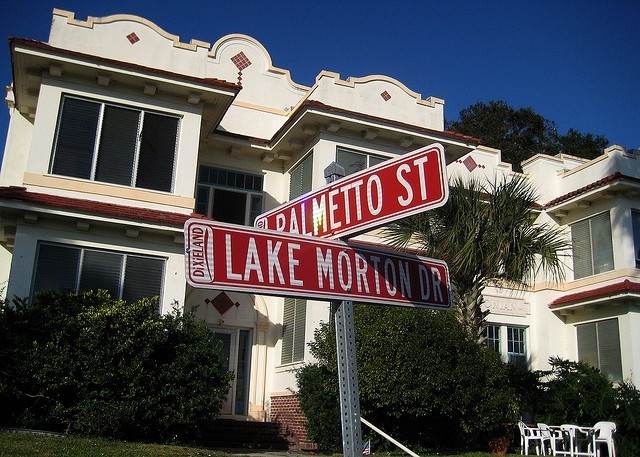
[519,422,617,457]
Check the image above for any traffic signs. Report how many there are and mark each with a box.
[253,139,450,240]
[183,216,451,312]
